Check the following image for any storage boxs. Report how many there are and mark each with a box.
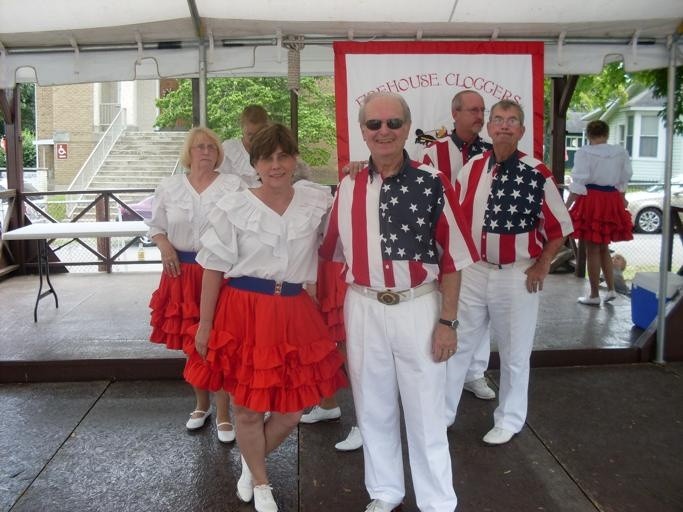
[631,271,683,333]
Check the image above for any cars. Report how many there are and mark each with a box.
[120,195,160,248]
[624,178,683,231]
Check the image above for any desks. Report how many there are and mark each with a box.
[1,221,153,323]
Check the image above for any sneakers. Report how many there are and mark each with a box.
[364,499,406,512]
[603,290,617,302]
[335,426,362,451]
[253,485,278,512]
[464,378,496,402]
[186,405,213,432]
[237,454,253,504]
[482,426,515,445]
[299,406,343,424]
[579,296,601,306]
[215,417,236,444]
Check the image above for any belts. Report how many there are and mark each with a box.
[477,260,536,271]
[351,282,436,305]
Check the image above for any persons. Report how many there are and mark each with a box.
[194,123,366,512]
[216,105,311,190]
[602,254,629,295]
[566,120,633,305]
[305,92,481,512]
[455,99,574,447]
[147,127,249,444]
[422,89,496,400]
[297,228,365,452]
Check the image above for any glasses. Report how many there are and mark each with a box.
[365,118,406,131]
[192,144,219,153]
[492,117,521,126]
[459,107,490,117]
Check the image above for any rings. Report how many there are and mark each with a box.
[170,261,175,266]
[450,350,455,354]
[532,280,537,284]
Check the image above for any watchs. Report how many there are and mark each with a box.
[438,318,460,330]
